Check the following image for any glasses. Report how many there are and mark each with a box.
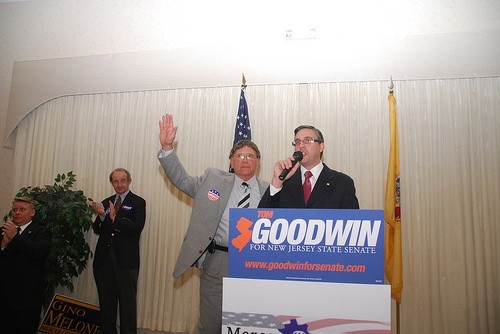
[233,153,258,161]
[292,136,322,146]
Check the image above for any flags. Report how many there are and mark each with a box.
[384,94,403,304]
[229,89,252,173]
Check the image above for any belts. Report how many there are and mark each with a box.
[215,244,228,252]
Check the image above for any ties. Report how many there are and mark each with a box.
[16,226,21,236]
[114,195,121,216]
[237,182,251,208]
[302,171,313,205]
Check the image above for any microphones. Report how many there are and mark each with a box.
[279,151,303,181]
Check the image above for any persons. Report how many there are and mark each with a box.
[157,114,269,334]
[0,196,50,334]
[92,168,147,333]
[257,125,360,209]
